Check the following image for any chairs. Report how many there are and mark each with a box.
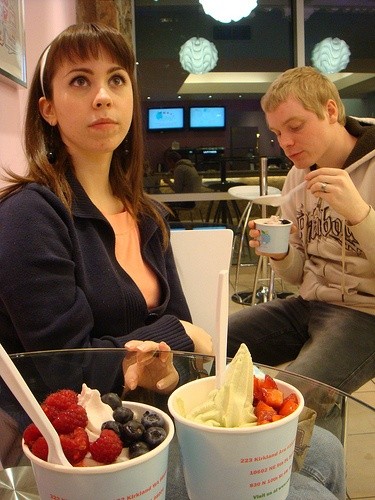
[169,229,233,348]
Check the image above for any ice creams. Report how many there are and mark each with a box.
[262,215,290,225]
[189,342,257,428]
[72,383,132,466]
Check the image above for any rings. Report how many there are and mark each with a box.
[320,183,327,192]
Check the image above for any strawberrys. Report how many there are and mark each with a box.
[253,375,298,428]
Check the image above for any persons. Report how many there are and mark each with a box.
[0,24,346,500]
[156,149,200,220]
[229,66,375,443]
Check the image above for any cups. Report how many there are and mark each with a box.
[166,375,305,500]
[253,218,293,254]
[21,401,175,500]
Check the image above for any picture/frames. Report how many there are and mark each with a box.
[0,0,28,90]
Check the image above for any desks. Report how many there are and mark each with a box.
[148,193,241,202]
[0,347,375,500]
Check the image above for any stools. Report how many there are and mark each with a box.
[229,186,284,306]
[202,180,252,262]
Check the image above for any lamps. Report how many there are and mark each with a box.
[199,0,258,23]
[311,37,351,74]
[178,3,218,74]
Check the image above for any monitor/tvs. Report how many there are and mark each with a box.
[188,106,226,130]
[146,107,186,131]
